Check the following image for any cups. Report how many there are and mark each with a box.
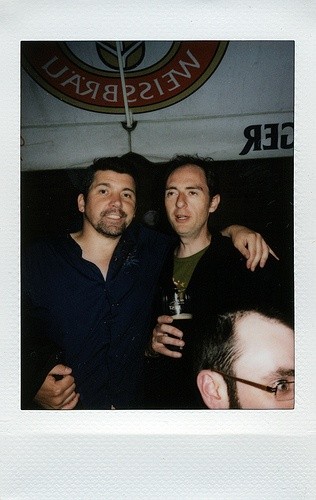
[161,288,194,352]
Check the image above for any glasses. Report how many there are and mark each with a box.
[210,366,295,401]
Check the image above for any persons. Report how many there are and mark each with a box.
[105,153,279,410]
[22,155,279,420]
[197,302,295,410]
